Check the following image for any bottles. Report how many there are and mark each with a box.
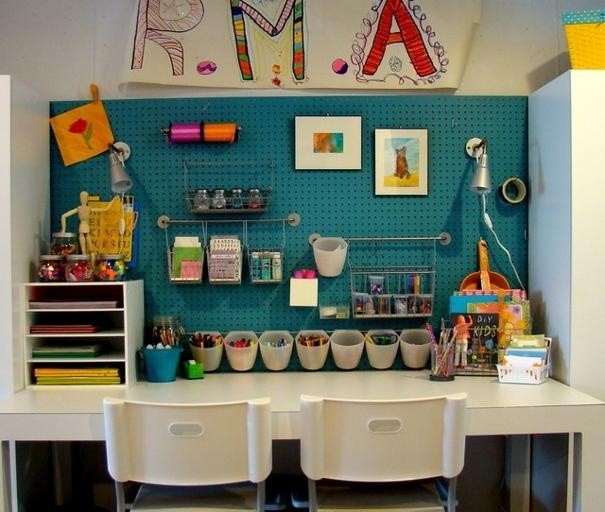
[95,255,125,281]
[194,190,211,209]
[50,233,79,255]
[211,190,226,208]
[230,189,243,209]
[249,189,260,209]
[38,255,65,282]
[65,255,94,281]
[151,314,184,347]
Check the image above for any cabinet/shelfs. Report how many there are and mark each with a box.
[24,279,144,387]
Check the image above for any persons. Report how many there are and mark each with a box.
[61,191,119,268]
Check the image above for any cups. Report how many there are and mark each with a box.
[430,344,456,382]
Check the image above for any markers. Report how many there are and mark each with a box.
[226,338,255,348]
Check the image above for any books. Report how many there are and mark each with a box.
[29,301,121,386]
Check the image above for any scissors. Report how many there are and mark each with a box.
[173,327,186,341]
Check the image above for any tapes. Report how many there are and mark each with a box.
[187,360,196,365]
[498,177,528,205]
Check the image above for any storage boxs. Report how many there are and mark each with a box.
[562,7,605,70]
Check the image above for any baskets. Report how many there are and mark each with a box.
[248,247,284,284]
[497,337,552,385]
[564,22,605,70]
[350,266,436,318]
[441,316,531,377]
[206,245,242,284]
[186,193,272,214]
[167,248,204,284]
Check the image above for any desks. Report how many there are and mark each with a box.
[1,371,603,512]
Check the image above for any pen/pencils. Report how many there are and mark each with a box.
[159,328,179,346]
[425,317,458,377]
[264,337,286,348]
[297,334,329,347]
[189,333,223,348]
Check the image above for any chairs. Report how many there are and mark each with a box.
[103,395,287,512]
[288,392,467,512]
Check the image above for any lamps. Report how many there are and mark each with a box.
[106,140,133,194]
[464,137,491,195]
